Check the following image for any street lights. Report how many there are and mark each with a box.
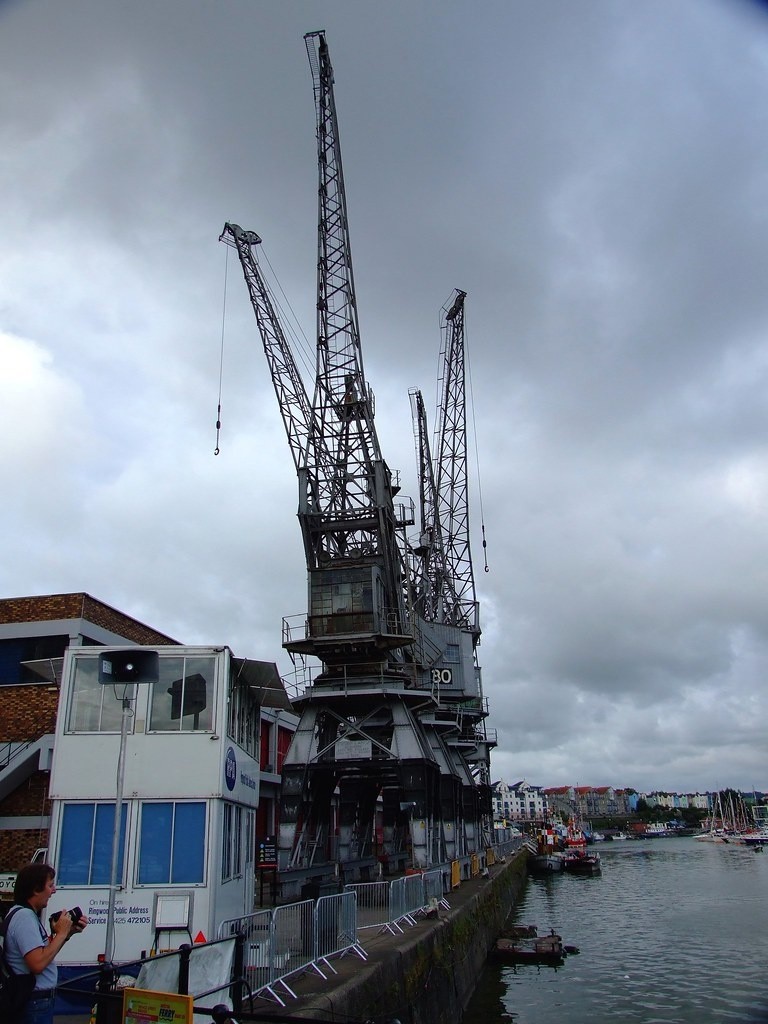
[94,647,158,1023]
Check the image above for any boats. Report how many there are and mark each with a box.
[627,830,640,840]
[645,821,685,838]
[609,832,627,840]
[531,809,605,874]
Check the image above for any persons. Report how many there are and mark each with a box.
[2,864,89,1024]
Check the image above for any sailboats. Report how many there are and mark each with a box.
[693,779,768,845]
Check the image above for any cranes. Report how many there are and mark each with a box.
[220,22,496,883]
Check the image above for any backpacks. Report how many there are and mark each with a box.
[0,907,45,1024]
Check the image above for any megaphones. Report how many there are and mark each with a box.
[98,650,160,683]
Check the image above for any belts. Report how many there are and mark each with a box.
[26,989,57,1001]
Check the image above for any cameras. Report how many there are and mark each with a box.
[50,907,82,926]
